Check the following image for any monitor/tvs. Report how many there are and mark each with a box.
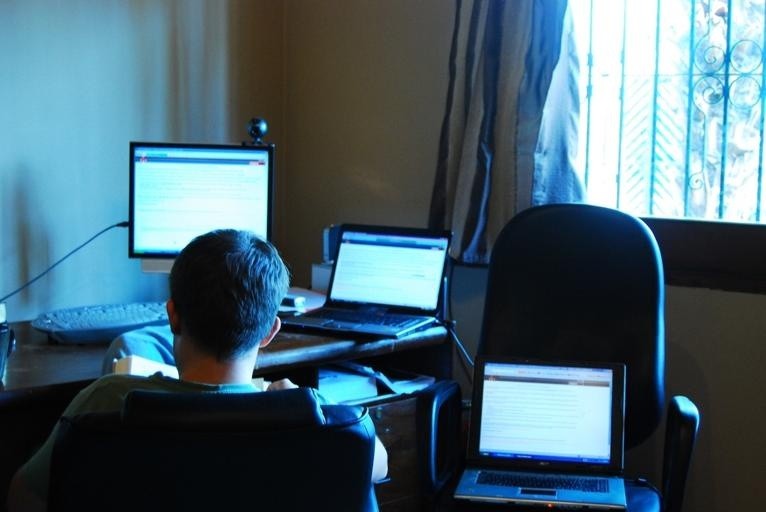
[127,140,277,261]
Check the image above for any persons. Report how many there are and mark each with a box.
[6,229,388,512]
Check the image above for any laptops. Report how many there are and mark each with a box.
[453,354,629,512]
[278,222,455,340]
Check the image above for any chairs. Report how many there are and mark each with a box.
[48,387,380,512]
[415,202,702,512]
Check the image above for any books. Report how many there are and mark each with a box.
[111,353,272,391]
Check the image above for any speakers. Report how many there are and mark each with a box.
[322,224,342,266]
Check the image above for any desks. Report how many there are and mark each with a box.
[1,321,453,511]
